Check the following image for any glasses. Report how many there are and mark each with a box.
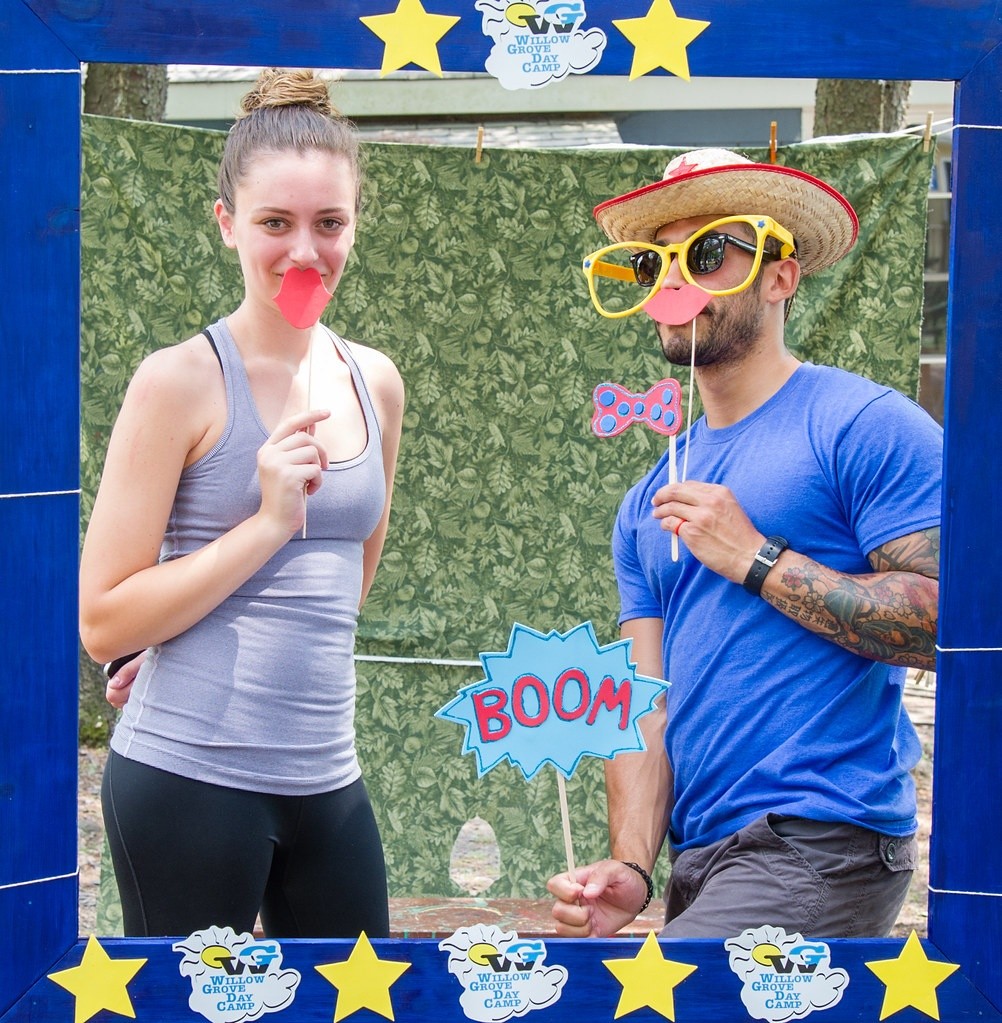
[627,231,782,287]
[581,215,796,319]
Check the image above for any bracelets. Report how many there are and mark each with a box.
[741,533,791,599]
[621,860,656,913]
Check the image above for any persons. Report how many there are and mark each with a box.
[76,62,416,938]
[548,167,947,946]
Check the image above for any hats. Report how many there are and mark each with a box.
[592,147,859,277]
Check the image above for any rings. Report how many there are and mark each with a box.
[674,517,686,537]
